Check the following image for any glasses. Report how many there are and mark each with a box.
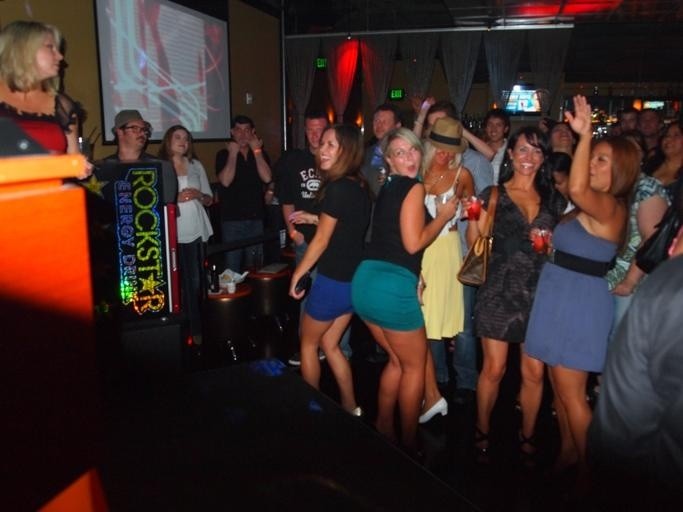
[123,125,152,134]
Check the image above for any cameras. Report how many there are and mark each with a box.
[587,123,614,143]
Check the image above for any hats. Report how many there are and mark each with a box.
[424,115,469,153]
[111,109,151,134]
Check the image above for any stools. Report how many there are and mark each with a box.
[199,284,257,361]
[279,250,296,272]
[240,267,293,337]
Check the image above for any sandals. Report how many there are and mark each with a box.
[515,429,539,475]
[470,425,493,471]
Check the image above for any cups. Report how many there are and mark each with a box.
[225,279,236,293]
[434,190,458,229]
[465,195,482,220]
[529,225,552,258]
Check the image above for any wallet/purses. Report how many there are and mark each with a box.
[294,271,313,300]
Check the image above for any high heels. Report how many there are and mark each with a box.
[418,397,449,424]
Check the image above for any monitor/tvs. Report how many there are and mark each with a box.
[500,84,542,116]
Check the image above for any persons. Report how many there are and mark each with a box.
[0,19,85,156]
[93,109,215,339]
[354,92,682,511]
[216,114,272,275]
[275,109,371,419]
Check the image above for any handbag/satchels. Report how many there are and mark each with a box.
[456,234,490,289]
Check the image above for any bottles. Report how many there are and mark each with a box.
[207,262,217,293]
